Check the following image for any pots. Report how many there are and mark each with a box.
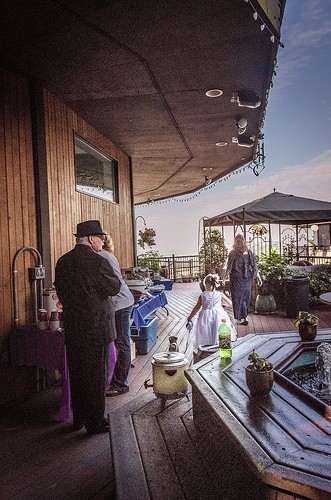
[151,351,189,398]
[42,287,63,310]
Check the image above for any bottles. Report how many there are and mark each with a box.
[218,319,232,358]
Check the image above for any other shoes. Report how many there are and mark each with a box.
[105,388,120,397]
[240,318,249,323]
[72,418,110,433]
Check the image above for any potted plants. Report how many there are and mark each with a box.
[254,249,331,342]
[244,349,274,397]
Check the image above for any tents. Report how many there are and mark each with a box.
[202,188,331,286]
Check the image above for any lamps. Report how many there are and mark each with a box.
[231,92,261,147]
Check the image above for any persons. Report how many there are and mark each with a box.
[187,274,237,348]
[55,220,122,433]
[96,230,134,397]
[224,235,262,326]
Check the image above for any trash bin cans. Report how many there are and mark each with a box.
[283,275,310,318]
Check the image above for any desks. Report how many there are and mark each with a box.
[130,290,169,330]
[18,323,118,422]
[151,276,172,291]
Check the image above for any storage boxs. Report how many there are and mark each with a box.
[130,314,158,355]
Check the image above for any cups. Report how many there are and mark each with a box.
[37,309,64,332]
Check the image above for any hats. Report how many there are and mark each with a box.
[73,220,107,237]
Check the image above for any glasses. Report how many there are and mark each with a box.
[95,235,104,241]
